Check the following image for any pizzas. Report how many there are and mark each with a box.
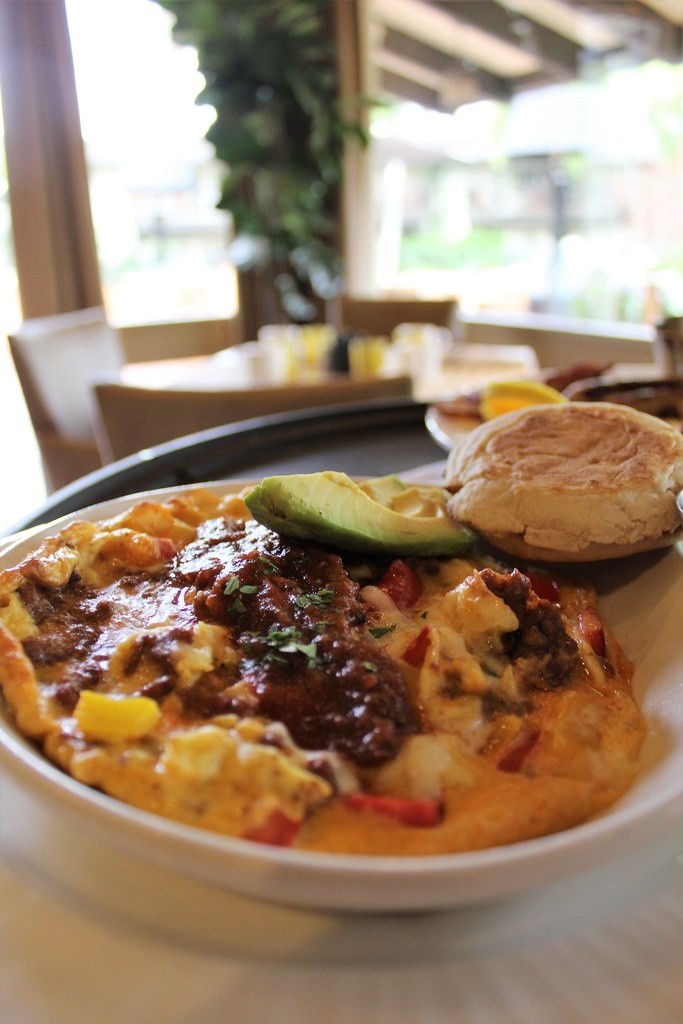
[0,469,650,855]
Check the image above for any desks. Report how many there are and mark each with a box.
[98,352,411,462]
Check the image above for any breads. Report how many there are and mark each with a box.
[440,400,682,562]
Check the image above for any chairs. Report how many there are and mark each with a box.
[7,305,125,496]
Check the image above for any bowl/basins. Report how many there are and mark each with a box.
[0,474,683,914]
